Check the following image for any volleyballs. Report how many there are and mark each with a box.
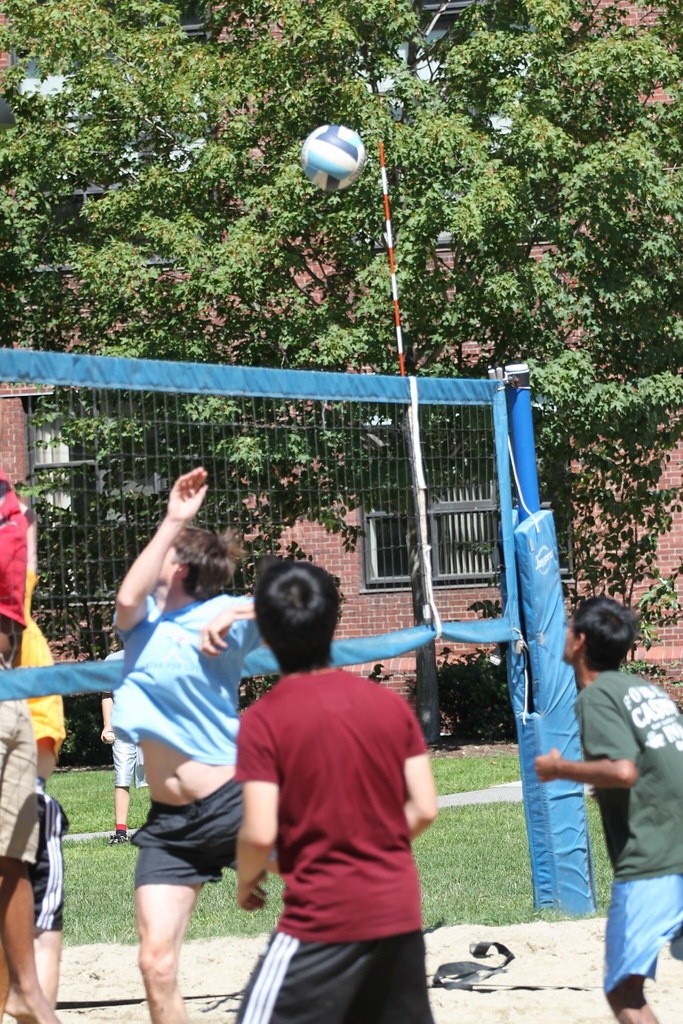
[300,124,366,193]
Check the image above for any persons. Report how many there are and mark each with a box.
[0,494,68,1022]
[534,601,683,1024]
[98,649,149,841]
[235,558,438,1024]
[0,474,67,1023]
[110,463,280,1023]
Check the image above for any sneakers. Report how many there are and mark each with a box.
[109,831,128,844]
